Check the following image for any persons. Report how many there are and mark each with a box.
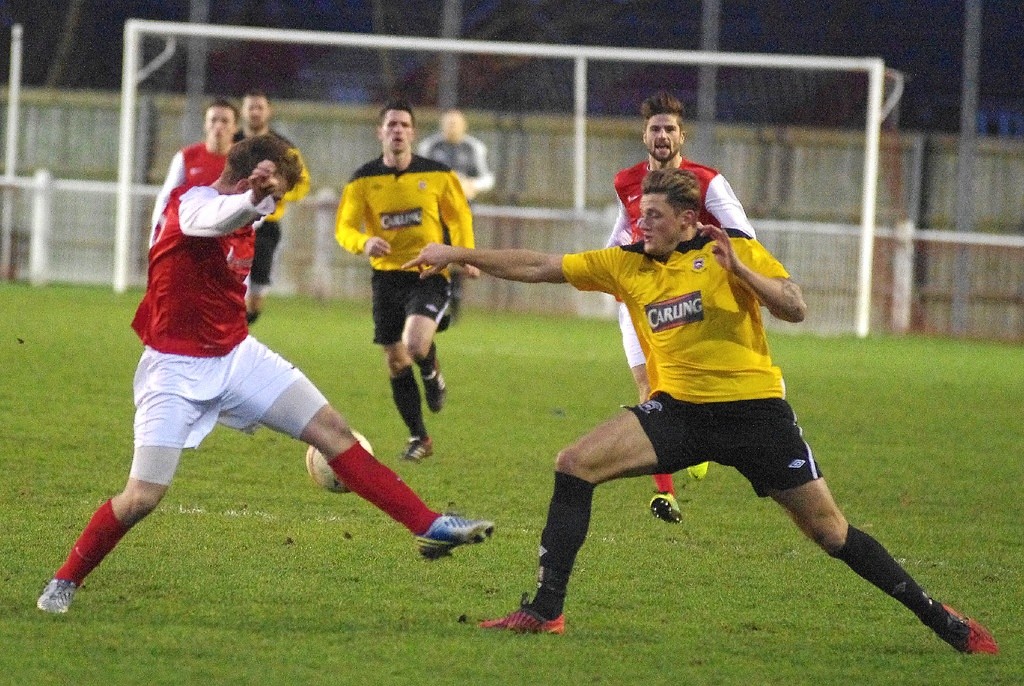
[232,88,310,325]
[417,109,494,201]
[334,98,478,462]
[149,99,242,248]
[35,136,494,614]
[400,168,998,654]
[602,92,757,522]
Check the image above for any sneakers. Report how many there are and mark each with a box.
[405,438,433,460]
[421,359,446,412]
[416,512,495,559]
[934,604,999,655]
[650,491,683,524]
[37,578,77,614]
[686,461,709,480]
[479,592,565,634]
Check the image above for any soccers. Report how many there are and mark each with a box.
[305,427,374,494]
